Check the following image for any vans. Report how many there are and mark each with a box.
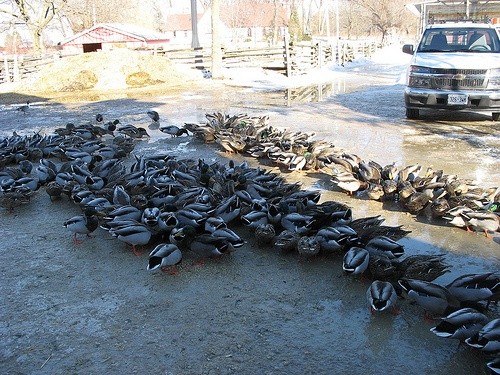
[402,23,500,119]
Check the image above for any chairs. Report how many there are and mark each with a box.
[468,33,487,50]
[430,33,448,47]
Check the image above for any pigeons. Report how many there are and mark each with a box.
[15,99,33,116]
[1,110,500,375]
